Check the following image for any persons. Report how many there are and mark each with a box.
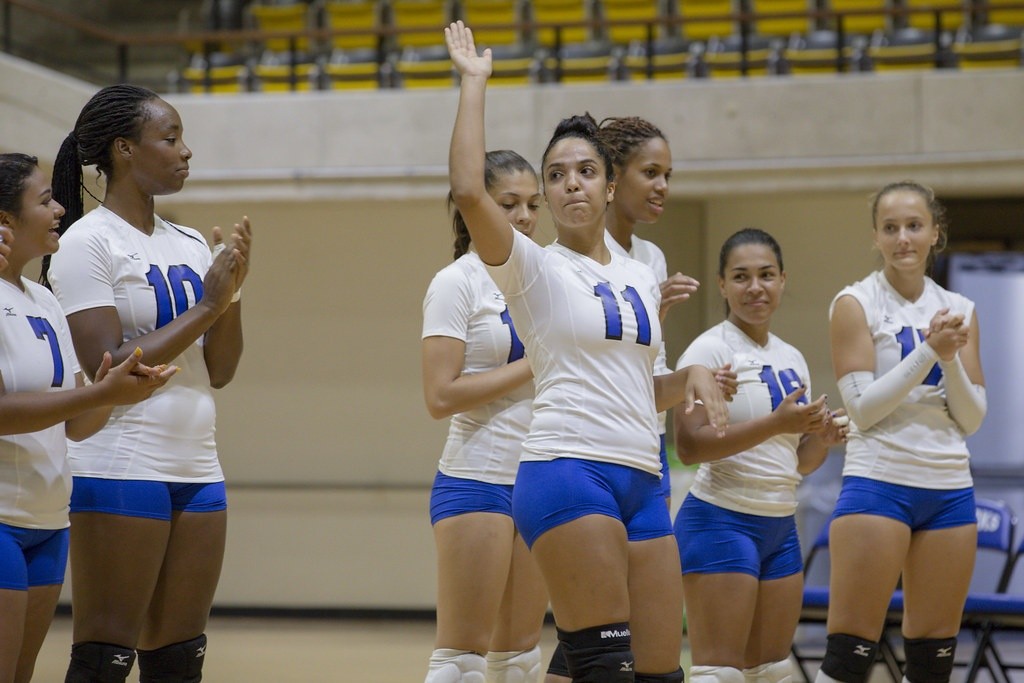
[544,116,740,683]
[420,149,548,683]
[445,20,730,683]
[0,87,252,683]
[815,182,986,683]
[673,228,850,683]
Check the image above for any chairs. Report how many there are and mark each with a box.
[176,0,1024,95]
[789,498,1024,683]
